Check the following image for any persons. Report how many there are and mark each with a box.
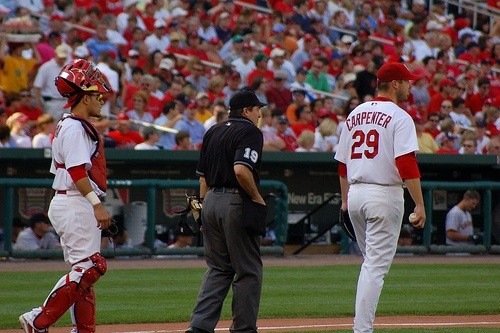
[15,214,60,250]
[102,221,132,260]
[155,223,195,257]
[186,90,269,333]
[19,58,111,333]
[334,61,426,333]
[0,0,499,153]
[446,190,481,256]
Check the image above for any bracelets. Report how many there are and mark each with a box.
[85,191,101,206]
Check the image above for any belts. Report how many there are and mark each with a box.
[215,186,239,192]
[57,190,66,194]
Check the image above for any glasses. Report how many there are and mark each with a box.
[87,93,103,101]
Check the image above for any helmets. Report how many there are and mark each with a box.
[55,59,113,108]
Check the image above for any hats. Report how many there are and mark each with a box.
[1,1,500,154]
[376,62,419,80]
[177,224,193,236]
[155,225,168,234]
[228,90,268,109]
[483,97,494,106]
[31,213,48,222]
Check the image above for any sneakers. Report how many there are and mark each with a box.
[71,326,78,333]
[19,315,47,333]
